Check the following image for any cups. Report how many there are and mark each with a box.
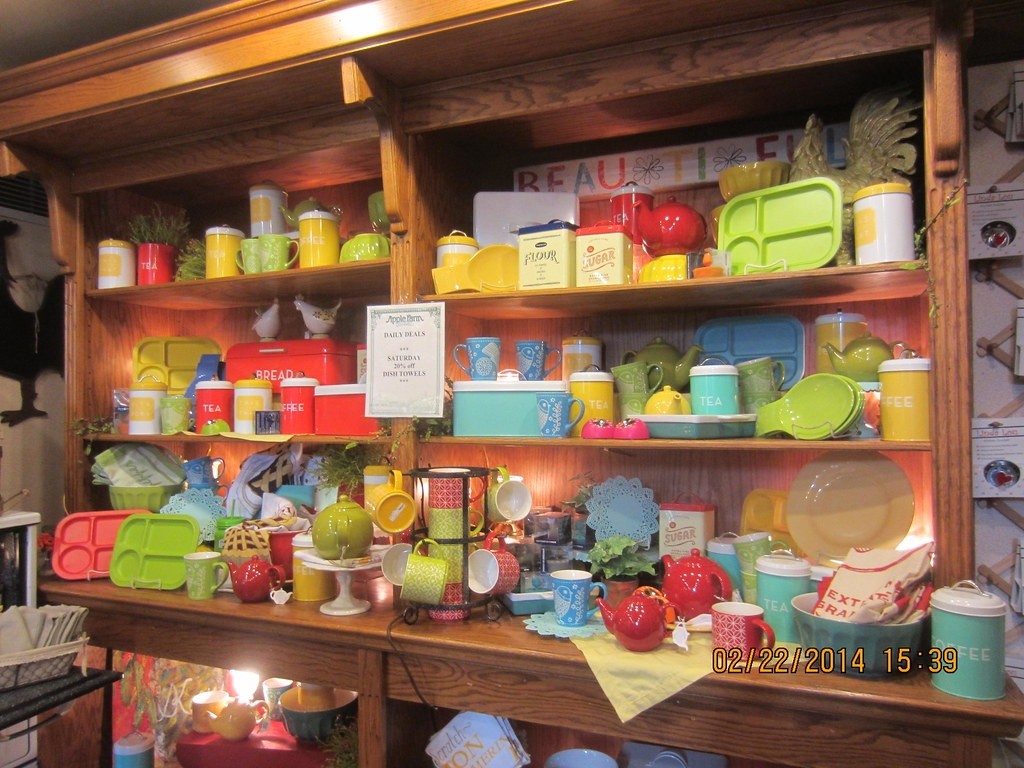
[813,309,933,442]
[98,238,136,290]
[113,730,157,768]
[129,375,319,435]
[437,228,480,269]
[203,180,341,280]
[852,182,916,265]
[181,457,1007,699]
[452,330,784,439]
[609,180,655,246]
[190,678,293,735]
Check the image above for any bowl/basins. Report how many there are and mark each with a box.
[718,160,790,203]
[638,255,688,285]
[277,687,358,745]
[338,234,392,262]
[368,188,390,229]
[109,485,185,514]
[791,593,925,678]
[543,748,619,768]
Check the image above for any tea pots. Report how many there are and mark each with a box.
[820,331,910,382]
[620,337,704,391]
[277,196,344,229]
[312,494,374,561]
[644,385,692,415]
[203,698,269,743]
[631,195,708,256]
[226,555,285,603]
[594,591,684,652]
[661,548,733,623]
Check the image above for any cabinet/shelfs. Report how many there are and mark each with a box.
[0,0,1024,768]
